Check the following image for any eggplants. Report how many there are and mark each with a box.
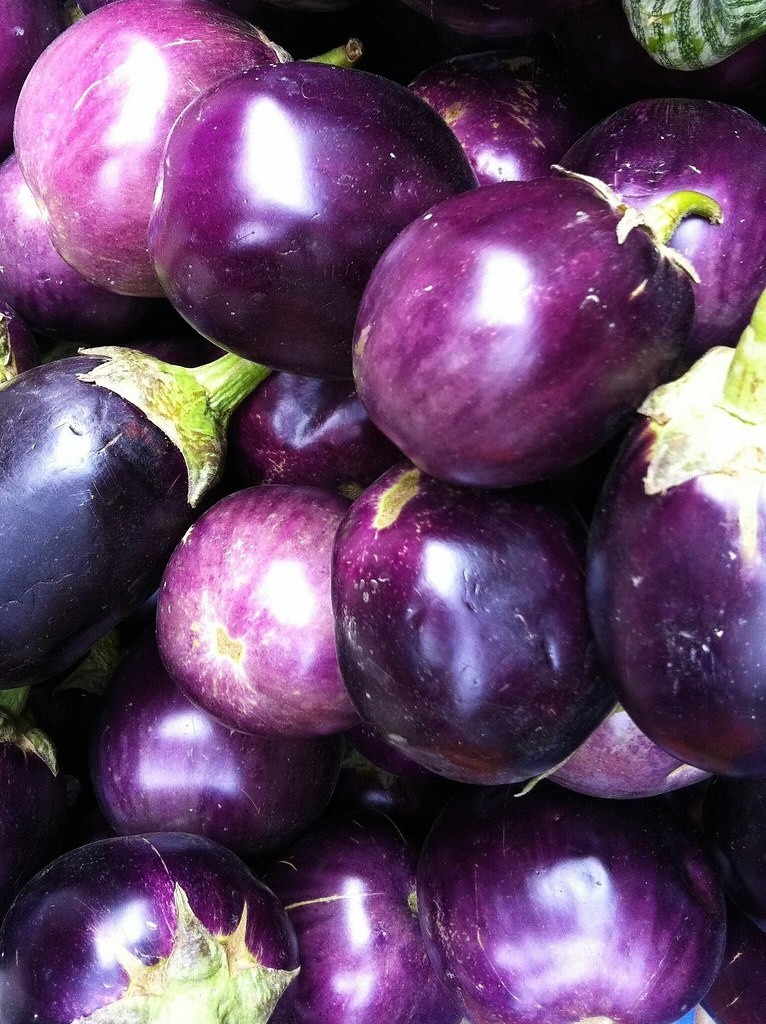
[1,0,764,1024]
